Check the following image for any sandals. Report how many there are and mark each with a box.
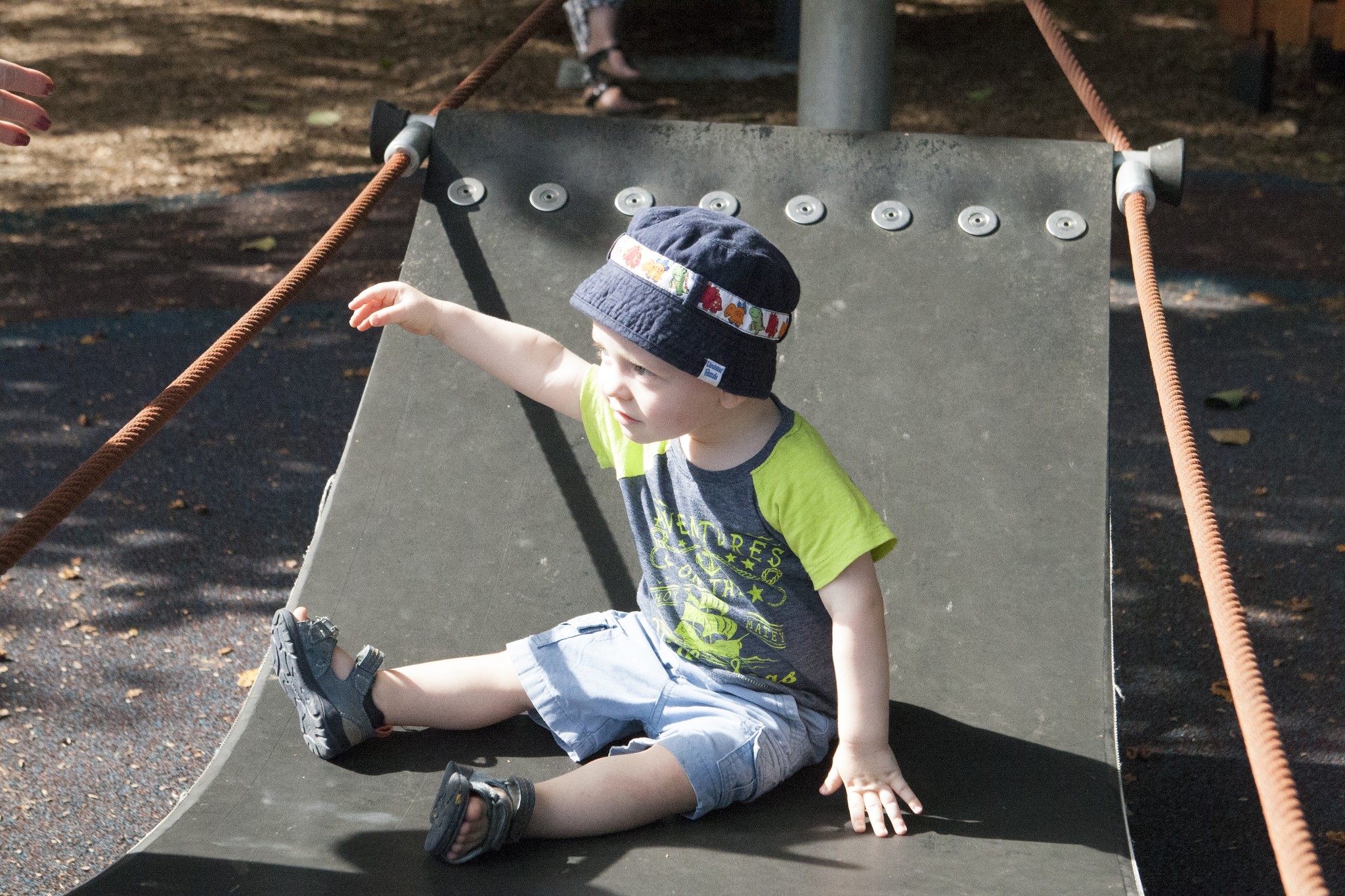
[576,82,649,114]
[424,760,535,863]
[270,607,394,760]
[584,44,640,82]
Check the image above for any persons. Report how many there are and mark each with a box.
[563,0,641,113]
[269,206,922,863]
[0,59,55,146]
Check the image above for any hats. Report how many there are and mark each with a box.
[570,209,801,401]
